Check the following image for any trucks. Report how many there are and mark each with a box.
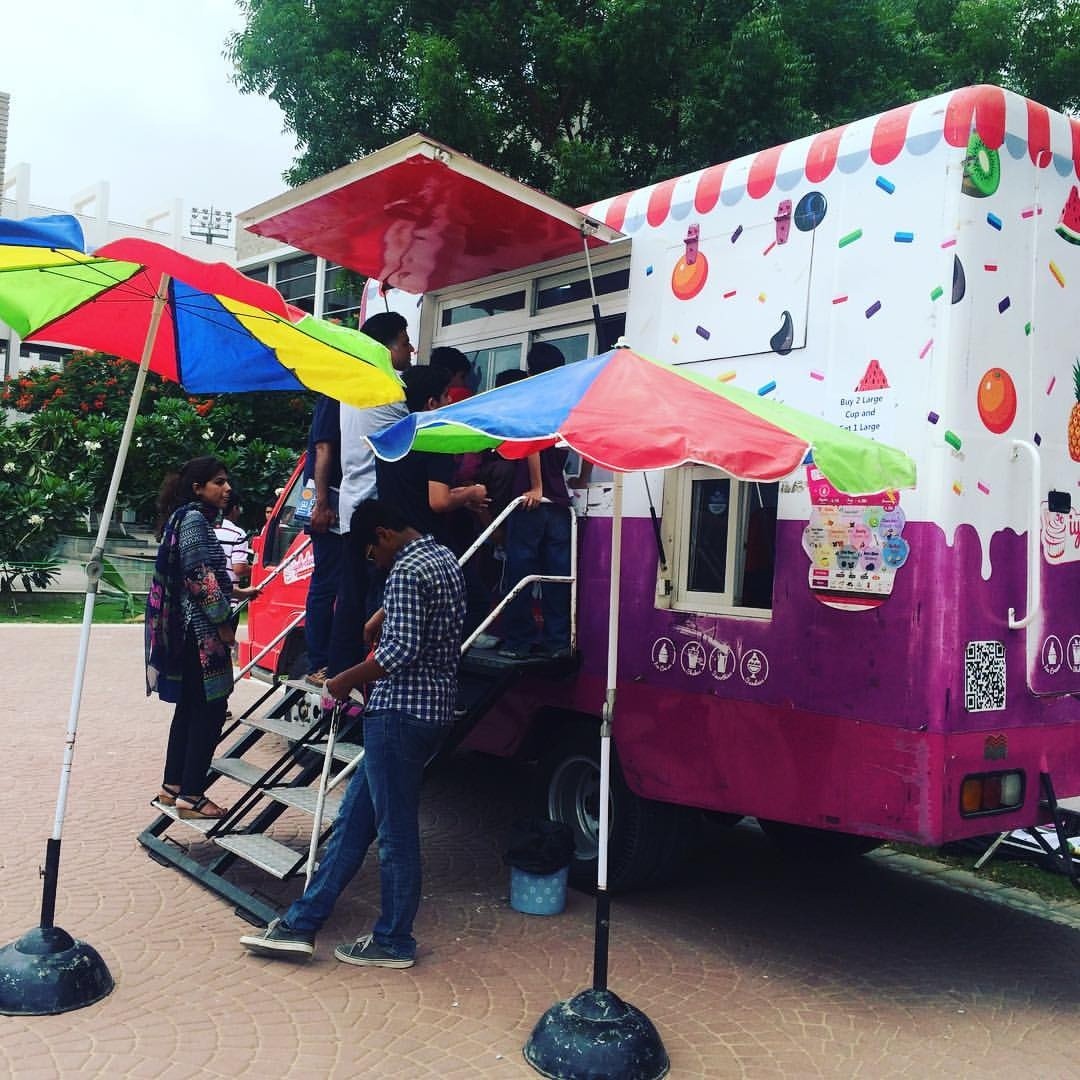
[223,83,1080,888]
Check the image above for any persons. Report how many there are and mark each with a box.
[145,458,274,821]
[291,312,593,686]
[238,502,468,968]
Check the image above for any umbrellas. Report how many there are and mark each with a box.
[358,337,919,890]
[0,214,404,836]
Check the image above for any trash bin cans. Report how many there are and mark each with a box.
[511,819,572,915]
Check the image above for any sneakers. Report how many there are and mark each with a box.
[335,934,416,969]
[239,917,314,957]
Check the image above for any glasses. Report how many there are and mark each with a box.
[365,543,376,560]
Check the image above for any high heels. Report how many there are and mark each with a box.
[158,784,182,805]
[176,794,227,819]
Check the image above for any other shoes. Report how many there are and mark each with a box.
[496,644,530,659]
[469,632,500,649]
[305,667,328,687]
[225,712,232,719]
[535,644,571,657]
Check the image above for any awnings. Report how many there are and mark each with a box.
[235,132,627,296]
